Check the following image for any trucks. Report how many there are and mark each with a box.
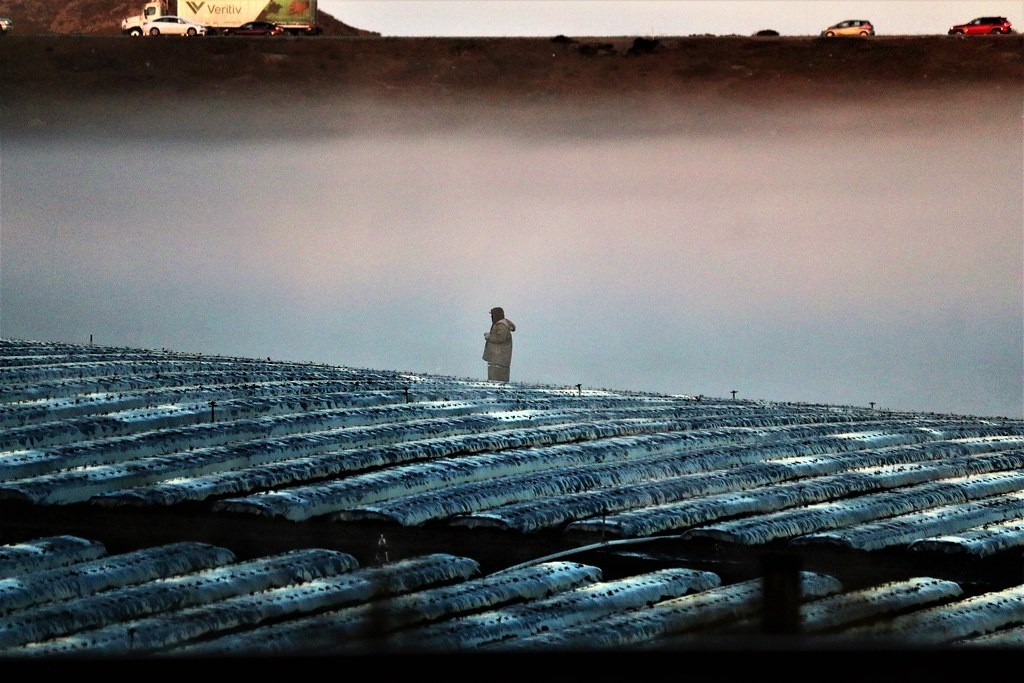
[121,0,317,34]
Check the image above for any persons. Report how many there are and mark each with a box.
[482,307,515,381]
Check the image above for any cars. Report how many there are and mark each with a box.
[0,17,14,34]
[143,15,207,36]
[822,20,875,37]
[223,21,284,36]
[948,16,1012,35]
